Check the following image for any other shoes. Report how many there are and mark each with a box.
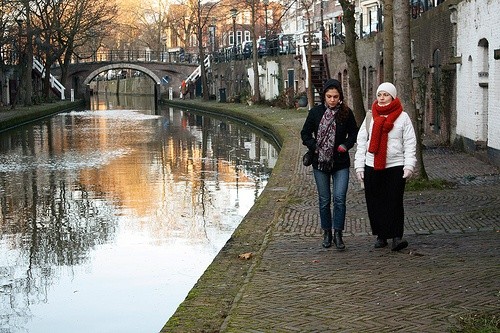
[391,237,409,251]
[374,238,388,248]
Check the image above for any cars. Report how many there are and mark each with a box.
[220,31,303,58]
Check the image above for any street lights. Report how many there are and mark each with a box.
[208,24,214,51]
[230,6,238,60]
[262,0,269,39]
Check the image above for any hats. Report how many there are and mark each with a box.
[376,82,397,100]
[323,79,344,104]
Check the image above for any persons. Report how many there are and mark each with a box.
[243,43,251,60]
[354,81,418,252]
[179,47,185,62]
[187,79,196,100]
[180,80,187,100]
[195,78,202,98]
[300,79,359,251]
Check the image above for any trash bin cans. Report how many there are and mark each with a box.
[217,87,226,103]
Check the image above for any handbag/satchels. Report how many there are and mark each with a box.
[303,149,315,166]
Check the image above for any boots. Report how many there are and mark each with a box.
[322,230,333,248]
[333,230,346,249]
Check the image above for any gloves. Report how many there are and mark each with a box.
[338,144,348,152]
[356,171,365,182]
[403,169,416,181]
